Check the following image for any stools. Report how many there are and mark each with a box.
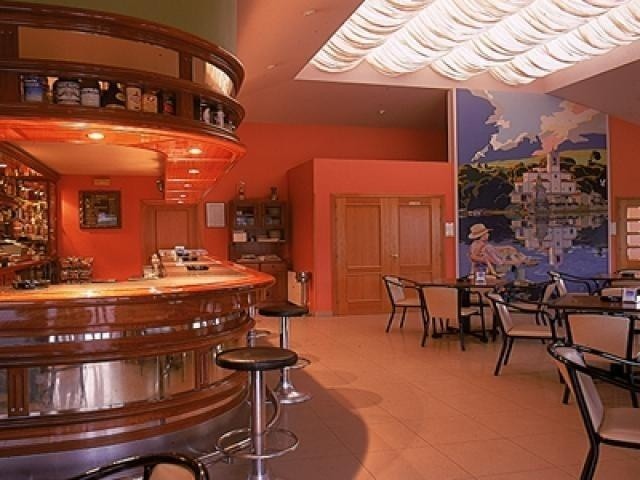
[211,345,299,479]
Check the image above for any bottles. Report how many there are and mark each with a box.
[238,182,248,201]
[271,185,279,202]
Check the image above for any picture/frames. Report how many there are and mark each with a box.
[79,190,124,230]
[451,89,612,290]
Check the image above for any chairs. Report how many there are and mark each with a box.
[463,273,498,306]
[564,312,637,409]
[259,268,312,405]
[383,275,420,333]
[414,282,484,350]
[507,280,556,344]
[485,292,565,376]
[548,342,639,480]
[550,270,612,295]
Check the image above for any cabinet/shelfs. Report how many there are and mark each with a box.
[0,142,63,285]
[0,1,247,208]
[227,200,292,307]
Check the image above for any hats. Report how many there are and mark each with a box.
[468,224,495,240]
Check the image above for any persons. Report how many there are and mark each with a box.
[467,223,541,284]
